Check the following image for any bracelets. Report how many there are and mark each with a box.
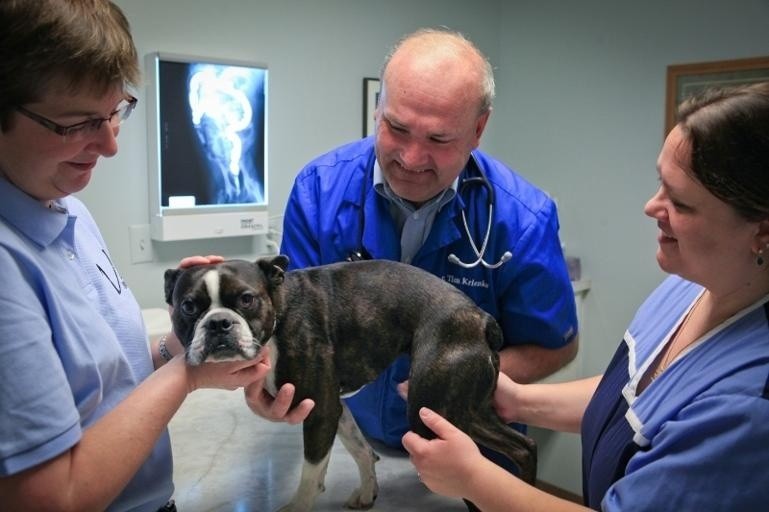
[159,333,173,362]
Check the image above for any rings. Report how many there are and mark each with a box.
[415,471,421,482]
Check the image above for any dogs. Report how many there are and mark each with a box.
[164,253,538,512]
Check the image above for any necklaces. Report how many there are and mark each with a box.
[648,292,712,382]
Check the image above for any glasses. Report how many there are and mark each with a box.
[16,91,137,144]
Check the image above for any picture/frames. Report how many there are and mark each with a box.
[362,77,383,139]
[664,57,769,140]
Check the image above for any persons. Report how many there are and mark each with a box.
[0,0,269,512]
[402,80,765,512]
[245,28,578,512]
